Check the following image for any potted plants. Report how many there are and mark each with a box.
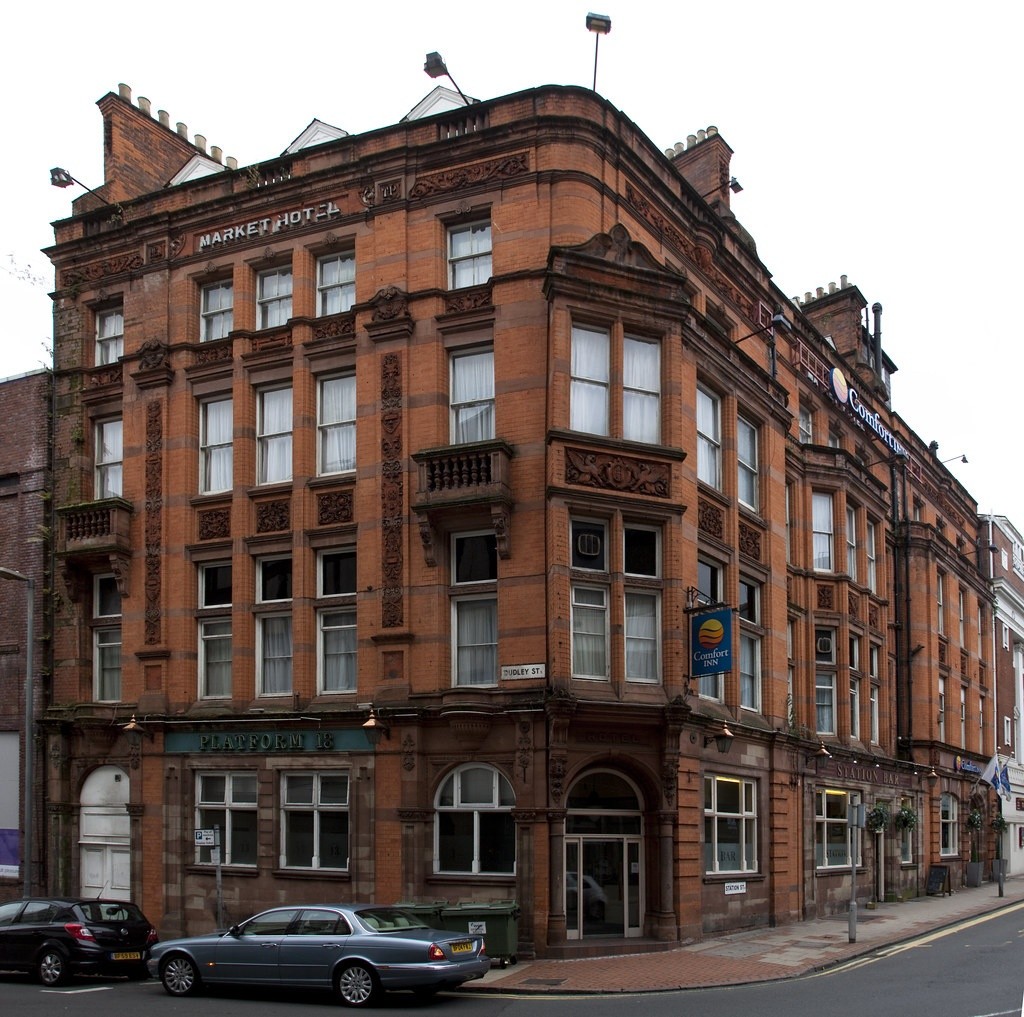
[987,842,1008,882]
[964,840,984,887]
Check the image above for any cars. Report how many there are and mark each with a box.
[1,897,159,987]
[148,902,493,1008]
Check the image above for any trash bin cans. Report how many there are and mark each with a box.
[441,898,522,970]
[389,900,449,929]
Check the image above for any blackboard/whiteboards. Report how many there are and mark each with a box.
[926,864,950,894]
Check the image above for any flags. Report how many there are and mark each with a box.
[999,765,1011,801]
[982,756,1002,799]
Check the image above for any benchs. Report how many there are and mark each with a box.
[365,917,409,928]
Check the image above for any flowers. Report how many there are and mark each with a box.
[990,812,1007,834]
[966,808,981,832]
[895,805,917,832]
[866,805,889,833]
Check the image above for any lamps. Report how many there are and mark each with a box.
[734,314,792,343]
[701,176,743,199]
[867,450,910,469]
[871,756,879,767]
[50,168,123,216]
[423,51,471,105]
[703,718,734,753]
[918,765,938,787]
[941,454,968,464]
[806,739,830,767]
[964,544,999,555]
[122,713,155,748]
[362,708,390,745]
[586,12,611,91]
[847,753,857,764]
